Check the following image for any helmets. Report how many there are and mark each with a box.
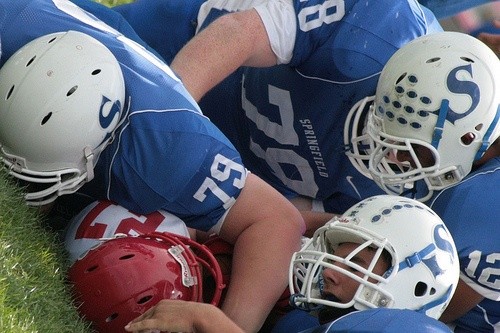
[288,195,460,320]
[66,232,224,333]
[365,32,500,204]
[0,30,125,206]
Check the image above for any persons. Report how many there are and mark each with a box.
[343,31,499,332]
[64,198,343,333]
[289,195,460,333]
[108,0,445,212]
[0,1,307,333]
